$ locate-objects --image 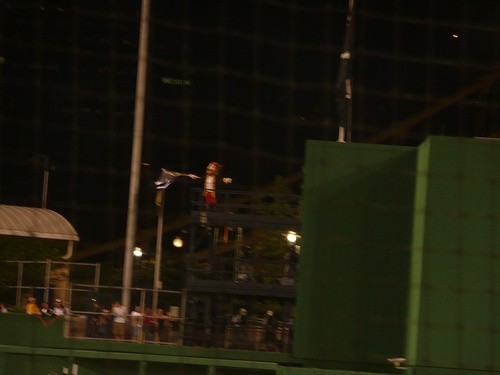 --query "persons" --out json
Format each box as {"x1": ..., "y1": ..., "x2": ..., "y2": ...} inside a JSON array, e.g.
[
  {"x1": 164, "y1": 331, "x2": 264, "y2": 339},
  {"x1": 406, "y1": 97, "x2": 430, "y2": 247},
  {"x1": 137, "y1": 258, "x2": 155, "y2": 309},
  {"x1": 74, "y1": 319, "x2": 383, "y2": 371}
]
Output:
[
  {"x1": 187, "y1": 162, "x2": 234, "y2": 210},
  {"x1": 233, "y1": 308, "x2": 294, "y2": 353},
  {"x1": 25, "y1": 296, "x2": 69, "y2": 317},
  {"x1": 88, "y1": 294, "x2": 180, "y2": 343}
]
